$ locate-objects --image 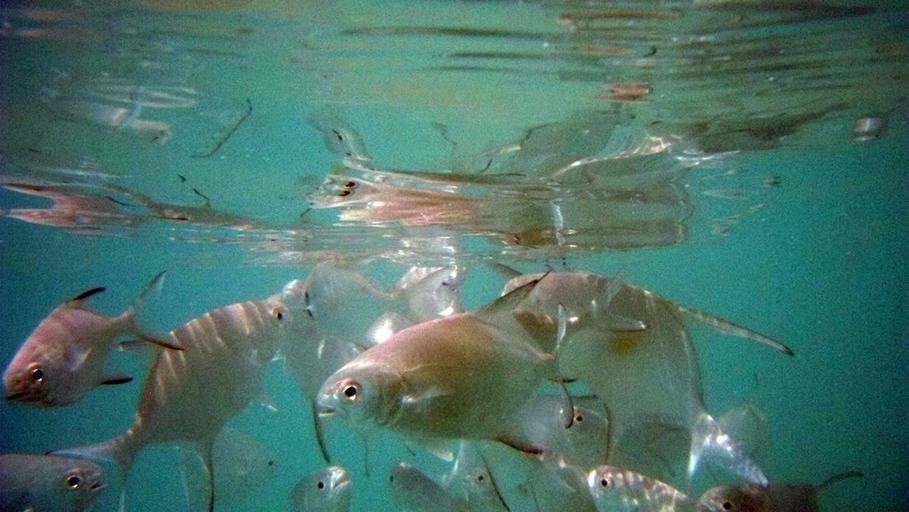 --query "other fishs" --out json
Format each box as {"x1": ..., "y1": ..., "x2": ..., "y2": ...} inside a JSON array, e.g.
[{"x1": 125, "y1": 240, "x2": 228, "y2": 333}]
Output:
[{"x1": 0, "y1": 247, "x2": 864, "y2": 511}]
